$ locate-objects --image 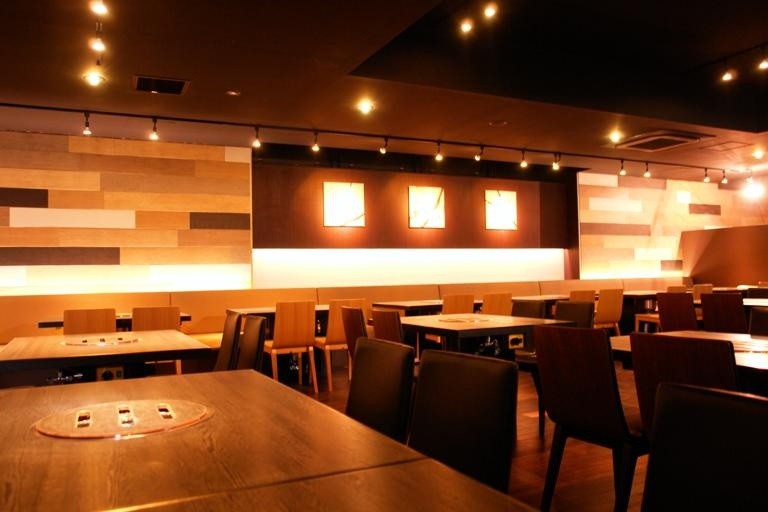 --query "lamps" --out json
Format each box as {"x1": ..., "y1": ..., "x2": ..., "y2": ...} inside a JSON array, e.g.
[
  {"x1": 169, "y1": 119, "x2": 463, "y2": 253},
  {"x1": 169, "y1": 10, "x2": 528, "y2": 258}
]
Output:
[{"x1": 68, "y1": 109, "x2": 729, "y2": 186}]
